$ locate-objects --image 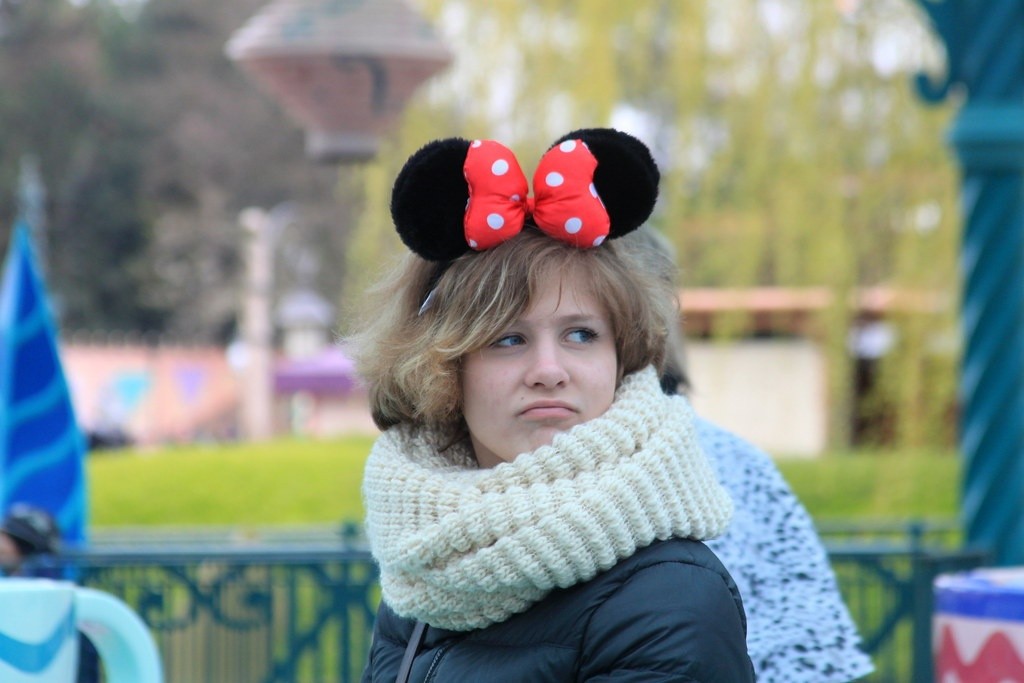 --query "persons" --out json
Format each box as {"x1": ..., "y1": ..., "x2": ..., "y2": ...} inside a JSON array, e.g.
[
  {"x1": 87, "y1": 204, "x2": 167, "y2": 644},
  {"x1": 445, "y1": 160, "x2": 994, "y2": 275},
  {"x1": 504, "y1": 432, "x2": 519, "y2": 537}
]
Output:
[{"x1": 365, "y1": 128, "x2": 874, "y2": 683}]
